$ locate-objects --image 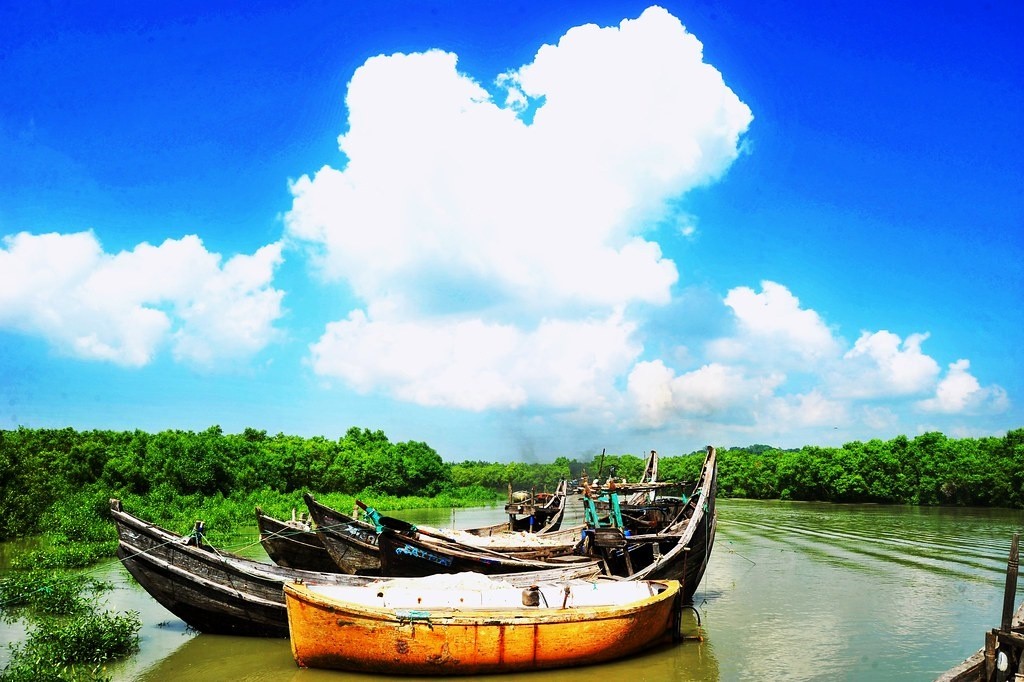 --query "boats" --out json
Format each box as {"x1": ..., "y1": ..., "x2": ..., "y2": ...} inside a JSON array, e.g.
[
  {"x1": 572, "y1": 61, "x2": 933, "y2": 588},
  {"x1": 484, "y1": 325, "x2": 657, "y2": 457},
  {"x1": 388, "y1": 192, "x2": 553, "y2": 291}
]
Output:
[
  {"x1": 109, "y1": 447, "x2": 718, "y2": 637},
  {"x1": 282, "y1": 570, "x2": 683, "y2": 674},
  {"x1": 929, "y1": 533, "x2": 1024, "y2": 682}
]
